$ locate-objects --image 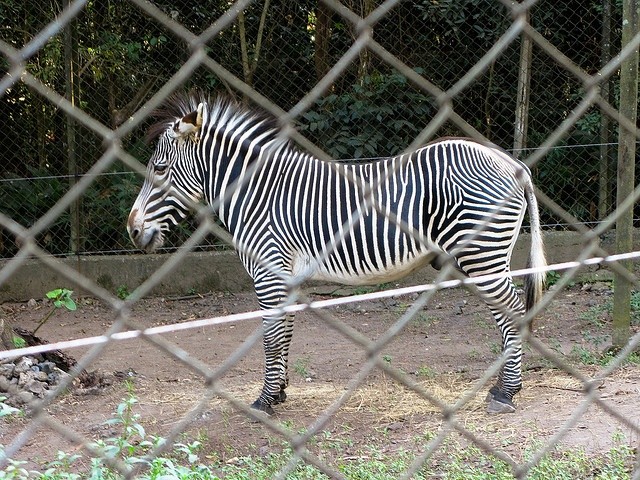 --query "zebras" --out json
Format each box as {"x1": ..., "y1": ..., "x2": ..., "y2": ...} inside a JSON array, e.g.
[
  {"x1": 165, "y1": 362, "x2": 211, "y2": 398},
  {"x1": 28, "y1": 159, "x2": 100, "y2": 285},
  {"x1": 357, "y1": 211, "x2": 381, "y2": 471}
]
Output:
[{"x1": 126, "y1": 87, "x2": 550, "y2": 424}]
